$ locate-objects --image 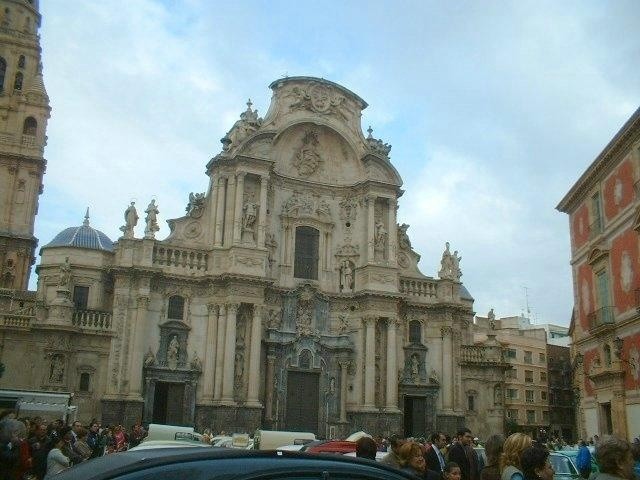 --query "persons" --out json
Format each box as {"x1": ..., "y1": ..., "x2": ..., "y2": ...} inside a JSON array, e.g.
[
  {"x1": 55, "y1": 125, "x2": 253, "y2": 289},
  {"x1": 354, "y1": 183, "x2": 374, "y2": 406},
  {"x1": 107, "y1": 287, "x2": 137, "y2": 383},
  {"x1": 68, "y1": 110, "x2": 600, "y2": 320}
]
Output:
[
  {"x1": 451, "y1": 251, "x2": 461, "y2": 274},
  {"x1": 355, "y1": 427, "x2": 640, "y2": 480},
  {"x1": 412, "y1": 356, "x2": 419, "y2": 374},
  {"x1": 243, "y1": 198, "x2": 257, "y2": 228},
  {"x1": 200, "y1": 428, "x2": 227, "y2": 444},
  {"x1": 58, "y1": 256, "x2": 72, "y2": 286},
  {"x1": 375, "y1": 218, "x2": 385, "y2": 247},
  {"x1": 124, "y1": 202, "x2": 139, "y2": 230},
  {"x1": 188, "y1": 192, "x2": 198, "y2": 215},
  {"x1": 0, "y1": 414, "x2": 149, "y2": 480},
  {"x1": 441, "y1": 242, "x2": 452, "y2": 272},
  {"x1": 341, "y1": 261, "x2": 352, "y2": 289},
  {"x1": 488, "y1": 309, "x2": 496, "y2": 330},
  {"x1": 167, "y1": 335, "x2": 180, "y2": 357},
  {"x1": 144, "y1": 199, "x2": 160, "y2": 230},
  {"x1": 51, "y1": 355, "x2": 63, "y2": 381}
]
